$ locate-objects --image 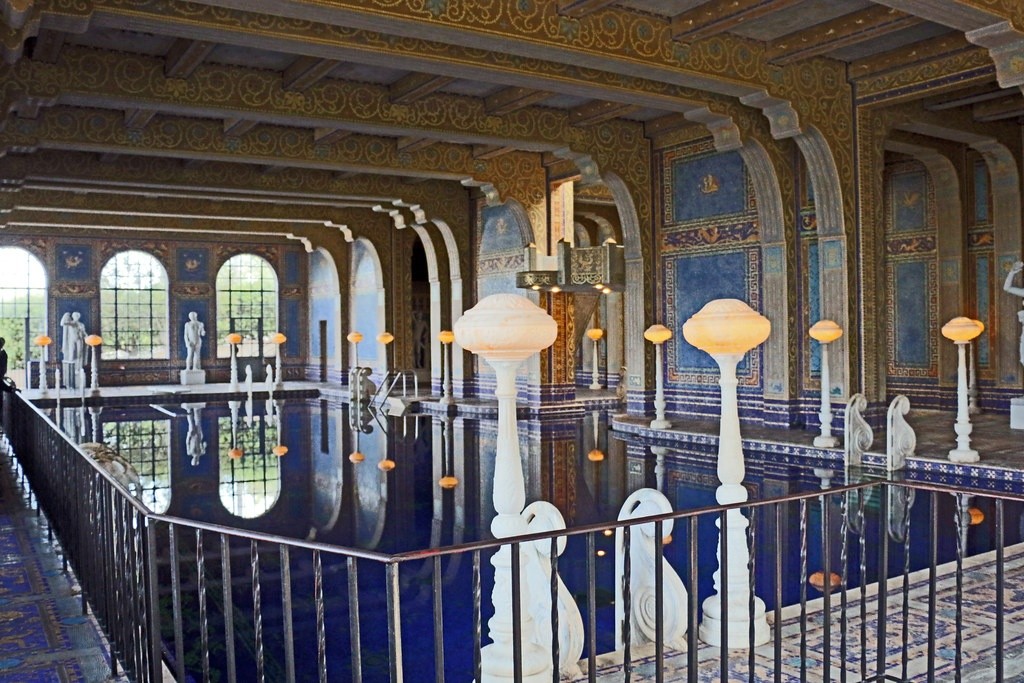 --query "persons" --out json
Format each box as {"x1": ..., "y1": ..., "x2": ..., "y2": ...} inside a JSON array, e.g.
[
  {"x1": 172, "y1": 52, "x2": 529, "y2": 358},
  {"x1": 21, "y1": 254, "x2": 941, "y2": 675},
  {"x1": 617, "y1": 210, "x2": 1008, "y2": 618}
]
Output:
[
  {"x1": 186, "y1": 407, "x2": 207, "y2": 466},
  {"x1": 0, "y1": 337, "x2": 7, "y2": 433},
  {"x1": 61, "y1": 312, "x2": 88, "y2": 363},
  {"x1": 184, "y1": 312, "x2": 206, "y2": 370}
]
